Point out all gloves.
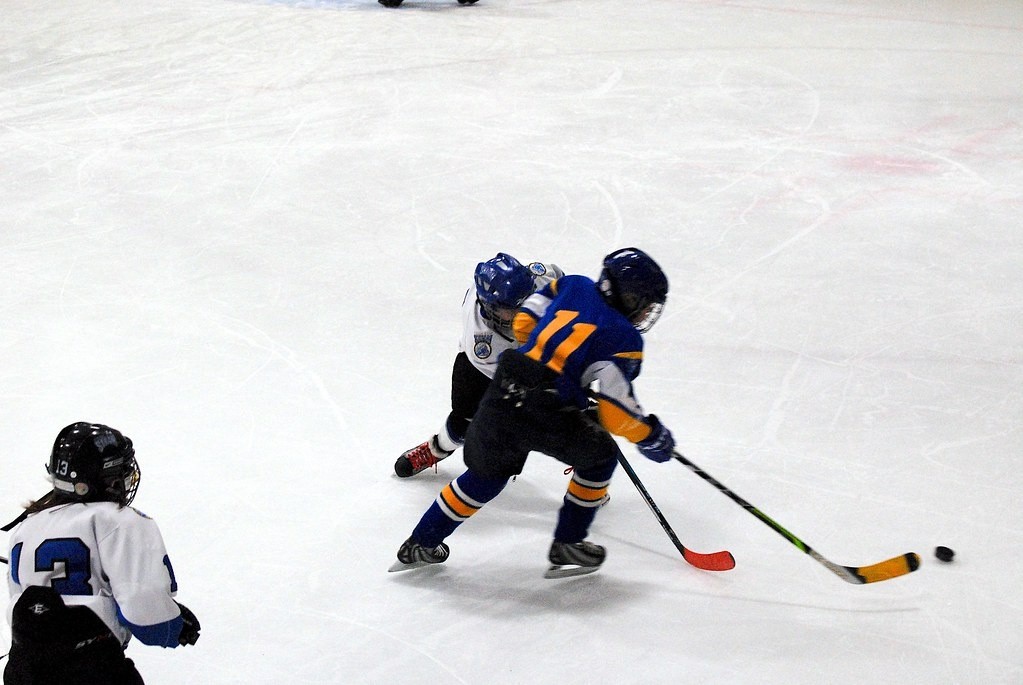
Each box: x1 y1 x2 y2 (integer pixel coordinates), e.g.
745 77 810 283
173 599 201 647
636 414 675 463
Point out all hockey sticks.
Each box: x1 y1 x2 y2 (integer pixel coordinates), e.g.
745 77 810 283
674 450 921 587
619 453 736 572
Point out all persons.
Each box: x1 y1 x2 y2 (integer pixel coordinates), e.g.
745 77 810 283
387 247 675 581
0 421 201 685
395 253 565 478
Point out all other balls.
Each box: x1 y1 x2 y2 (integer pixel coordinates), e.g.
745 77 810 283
935 545 955 562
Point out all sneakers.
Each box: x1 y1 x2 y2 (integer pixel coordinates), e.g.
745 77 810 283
394 434 454 477
388 536 450 571
544 540 606 579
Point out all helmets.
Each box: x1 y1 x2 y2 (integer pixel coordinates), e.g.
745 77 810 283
46 422 141 508
473 253 536 331
602 248 668 335
